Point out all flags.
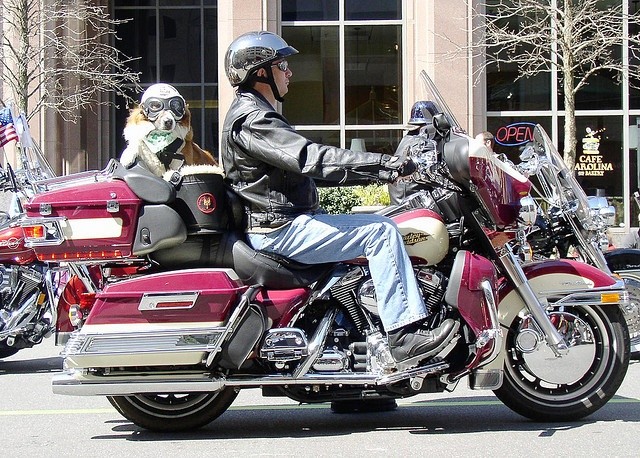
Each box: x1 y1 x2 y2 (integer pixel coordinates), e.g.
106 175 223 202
0 106 19 148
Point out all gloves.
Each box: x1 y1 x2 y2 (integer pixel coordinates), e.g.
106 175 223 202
380 153 416 176
378 170 397 184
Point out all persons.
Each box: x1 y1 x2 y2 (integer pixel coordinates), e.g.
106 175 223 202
221 29 461 414
387 101 445 206
475 132 495 150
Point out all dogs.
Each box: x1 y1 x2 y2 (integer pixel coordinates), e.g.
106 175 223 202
119 83 218 182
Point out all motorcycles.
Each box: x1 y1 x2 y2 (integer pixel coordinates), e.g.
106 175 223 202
510 123 640 360
21 69 631 432
0 160 146 358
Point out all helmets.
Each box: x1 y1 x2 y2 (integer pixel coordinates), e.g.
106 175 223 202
224 31 299 87
141 83 185 104
407 101 442 124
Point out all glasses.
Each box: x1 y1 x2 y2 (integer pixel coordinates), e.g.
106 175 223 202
271 59 289 71
141 96 185 117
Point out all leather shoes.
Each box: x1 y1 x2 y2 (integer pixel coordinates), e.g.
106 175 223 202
388 317 461 372
331 398 398 413
